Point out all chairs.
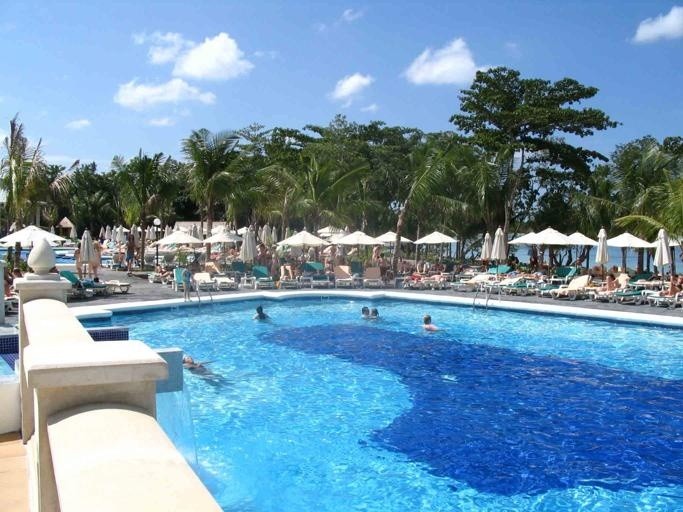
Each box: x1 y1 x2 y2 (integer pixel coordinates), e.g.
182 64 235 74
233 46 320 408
3 270 131 313
148 253 683 310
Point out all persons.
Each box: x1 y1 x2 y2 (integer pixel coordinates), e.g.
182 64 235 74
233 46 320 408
509 254 683 298
359 307 369 320
421 315 440 332
4 236 103 297
181 267 196 302
113 236 466 290
182 353 235 394
367 308 379 319
251 305 270 319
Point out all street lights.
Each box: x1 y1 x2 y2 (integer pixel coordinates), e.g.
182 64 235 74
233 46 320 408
153 217 161 266
